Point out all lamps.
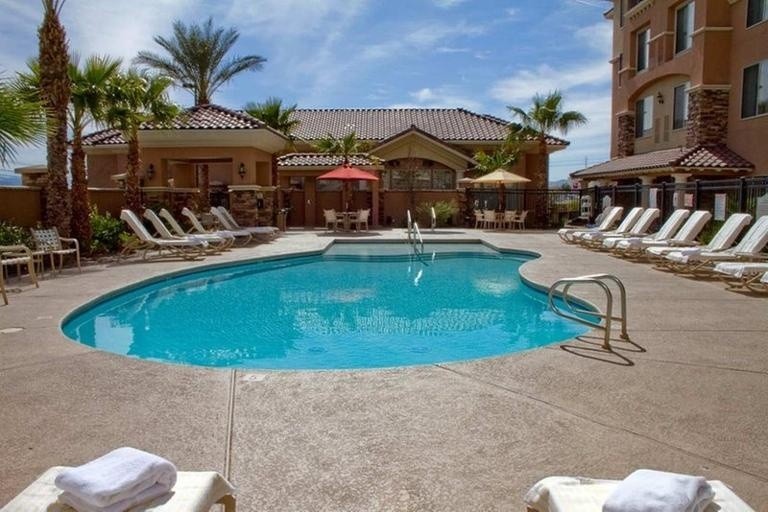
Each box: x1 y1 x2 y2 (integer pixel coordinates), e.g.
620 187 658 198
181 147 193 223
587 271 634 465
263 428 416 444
656 91 664 104
146 163 154 181
239 162 246 179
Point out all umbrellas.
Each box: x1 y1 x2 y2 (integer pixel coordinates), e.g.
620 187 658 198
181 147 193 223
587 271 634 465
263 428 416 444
316 161 380 212
470 168 532 209
471 273 521 298
328 286 371 302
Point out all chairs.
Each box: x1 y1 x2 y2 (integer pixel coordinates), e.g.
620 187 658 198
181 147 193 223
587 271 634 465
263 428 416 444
524 475 759 512
323 208 371 233
0 206 280 305
473 207 530 231
0 465 236 512
557 206 768 295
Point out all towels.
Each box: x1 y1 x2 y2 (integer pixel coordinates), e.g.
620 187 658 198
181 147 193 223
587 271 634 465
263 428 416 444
602 469 721 512
55 446 177 511
